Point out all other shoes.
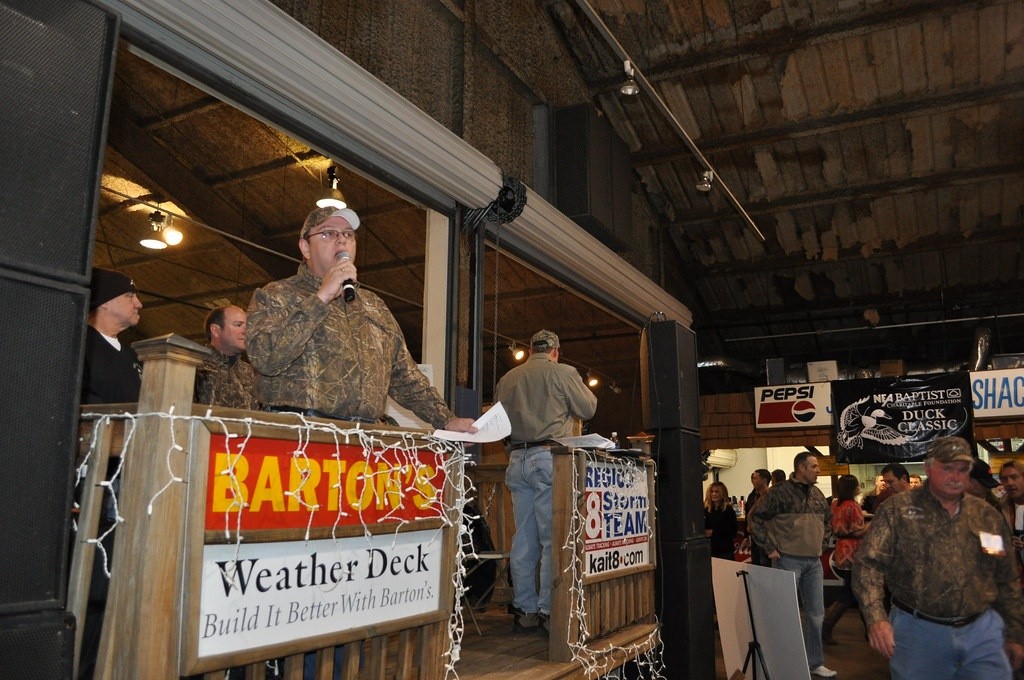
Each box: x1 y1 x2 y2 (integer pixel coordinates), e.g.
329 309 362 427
811 664 837 678
539 614 551 635
514 610 539 633
820 624 840 647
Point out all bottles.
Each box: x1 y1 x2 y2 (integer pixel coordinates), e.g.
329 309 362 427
610 432 620 449
728 496 746 517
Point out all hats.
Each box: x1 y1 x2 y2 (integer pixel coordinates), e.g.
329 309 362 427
90 264 138 310
531 329 561 355
969 459 1001 488
300 206 360 234
930 437 976 464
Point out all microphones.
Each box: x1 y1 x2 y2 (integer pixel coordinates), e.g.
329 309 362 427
334 251 355 301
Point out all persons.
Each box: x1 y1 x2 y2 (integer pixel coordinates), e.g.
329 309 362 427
851 438 1024 680
73 273 144 527
882 463 910 497
967 459 1024 581
705 482 739 560
748 453 837 677
823 474 870 643
910 474 922 487
245 207 478 680
745 469 786 567
497 329 596 636
194 306 261 412
861 474 885 522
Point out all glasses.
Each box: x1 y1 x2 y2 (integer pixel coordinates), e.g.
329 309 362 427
305 230 359 244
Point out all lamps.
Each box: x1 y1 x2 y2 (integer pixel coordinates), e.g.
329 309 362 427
508 341 524 360
696 170 713 191
585 369 598 387
327 165 343 200
609 380 621 393
620 60 639 95
163 214 184 246
140 211 167 250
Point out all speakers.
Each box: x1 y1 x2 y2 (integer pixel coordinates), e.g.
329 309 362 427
647 320 716 680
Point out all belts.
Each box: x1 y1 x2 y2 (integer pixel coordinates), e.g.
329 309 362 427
510 442 561 453
275 404 344 420
891 595 985 628
